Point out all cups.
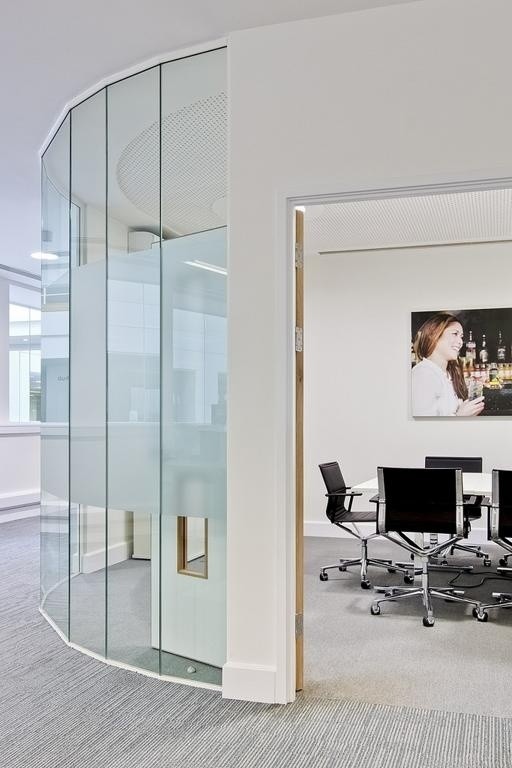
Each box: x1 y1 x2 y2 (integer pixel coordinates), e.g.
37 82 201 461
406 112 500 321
469 377 483 400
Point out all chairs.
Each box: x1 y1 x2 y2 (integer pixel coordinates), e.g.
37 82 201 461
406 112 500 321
319 456 512 626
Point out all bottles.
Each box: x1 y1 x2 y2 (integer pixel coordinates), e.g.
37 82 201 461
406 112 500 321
411 340 416 367
464 329 507 362
460 359 512 388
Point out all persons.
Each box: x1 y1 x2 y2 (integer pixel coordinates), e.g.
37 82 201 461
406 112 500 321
411 313 485 416
